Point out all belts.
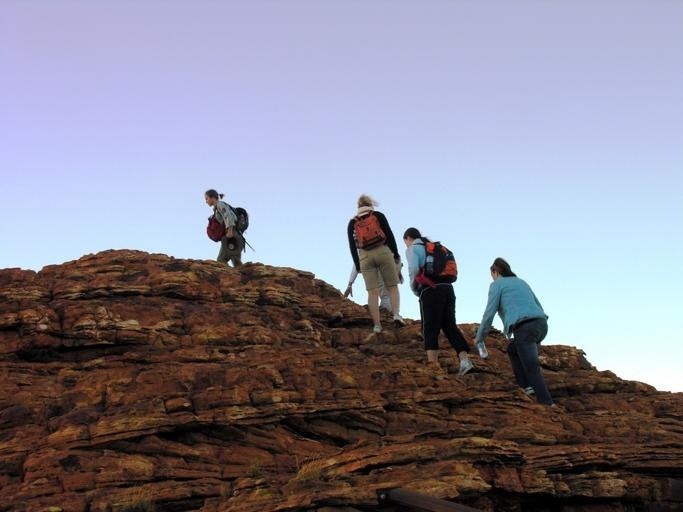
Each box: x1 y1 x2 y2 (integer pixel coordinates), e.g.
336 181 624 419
513 317 540 333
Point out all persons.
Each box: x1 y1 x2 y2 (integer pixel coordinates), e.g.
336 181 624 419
204 189 245 267
402 227 475 377
346 194 408 334
471 256 558 408
341 254 405 314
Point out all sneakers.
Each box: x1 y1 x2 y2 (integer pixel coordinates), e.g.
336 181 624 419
373 325 382 333
524 386 535 395
458 358 474 376
394 316 406 327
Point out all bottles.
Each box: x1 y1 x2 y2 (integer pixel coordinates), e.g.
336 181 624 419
426 254 434 275
477 340 488 358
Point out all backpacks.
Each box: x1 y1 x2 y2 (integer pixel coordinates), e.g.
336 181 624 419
413 241 458 284
206 205 226 242
354 211 386 250
224 202 250 234
